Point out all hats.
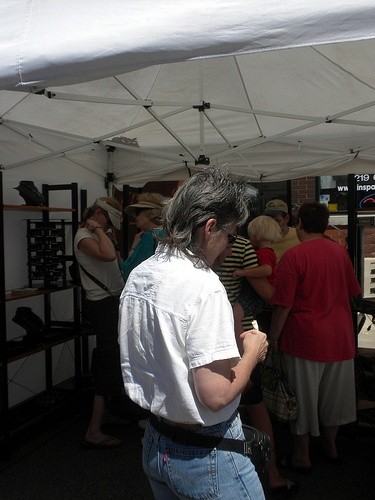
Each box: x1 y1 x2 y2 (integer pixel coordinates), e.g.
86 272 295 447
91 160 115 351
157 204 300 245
130 192 168 209
95 198 124 230
263 199 288 214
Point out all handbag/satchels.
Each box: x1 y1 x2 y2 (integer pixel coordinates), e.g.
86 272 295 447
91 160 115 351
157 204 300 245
261 353 298 428
242 423 276 468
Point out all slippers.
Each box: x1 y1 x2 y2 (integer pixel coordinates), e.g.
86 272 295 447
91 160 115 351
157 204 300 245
270 479 300 500
80 433 125 450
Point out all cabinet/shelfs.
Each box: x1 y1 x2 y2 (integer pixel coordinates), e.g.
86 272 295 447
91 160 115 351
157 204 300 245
0 168 87 463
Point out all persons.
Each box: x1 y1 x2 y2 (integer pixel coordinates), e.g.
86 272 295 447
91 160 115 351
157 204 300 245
117 165 269 500
74 192 362 494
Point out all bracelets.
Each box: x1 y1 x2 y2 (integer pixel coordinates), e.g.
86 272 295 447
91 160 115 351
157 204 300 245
94 226 100 230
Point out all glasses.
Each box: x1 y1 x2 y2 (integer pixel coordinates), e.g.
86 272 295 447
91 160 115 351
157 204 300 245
216 221 237 244
